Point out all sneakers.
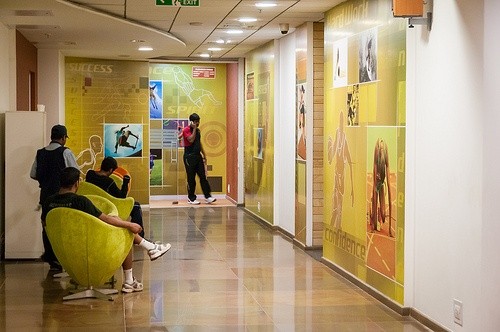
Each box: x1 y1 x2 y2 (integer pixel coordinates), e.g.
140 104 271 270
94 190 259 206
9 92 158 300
148 241 172 260
188 199 200 205
121 276 143 293
204 197 217 204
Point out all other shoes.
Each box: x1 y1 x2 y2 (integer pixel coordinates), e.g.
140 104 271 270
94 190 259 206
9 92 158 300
370 207 372 228
49 262 63 271
378 207 383 229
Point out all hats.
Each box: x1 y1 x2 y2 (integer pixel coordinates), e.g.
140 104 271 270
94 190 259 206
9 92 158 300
51 124 69 139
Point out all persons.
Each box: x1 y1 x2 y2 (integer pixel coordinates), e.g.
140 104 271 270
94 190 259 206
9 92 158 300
113 125 139 153
183 113 216 205
150 84 158 109
150 153 157 174
41 167 171 292
328 112 353 227
370 138 393 237
30 124 80 269
85 157 144 240
298 86 306 144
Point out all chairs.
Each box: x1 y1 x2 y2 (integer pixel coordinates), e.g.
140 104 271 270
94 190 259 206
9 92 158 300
44 166 135 302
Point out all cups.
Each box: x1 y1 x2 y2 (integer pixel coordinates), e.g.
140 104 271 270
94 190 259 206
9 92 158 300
38 104 45 112
